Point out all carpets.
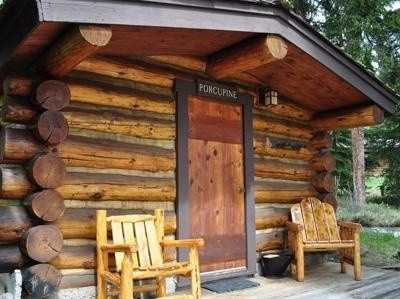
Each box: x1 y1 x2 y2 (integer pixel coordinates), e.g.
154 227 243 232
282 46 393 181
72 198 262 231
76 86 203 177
201 277 260 293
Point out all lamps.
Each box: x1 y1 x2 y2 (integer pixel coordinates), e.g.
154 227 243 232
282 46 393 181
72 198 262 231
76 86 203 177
259 86 278 106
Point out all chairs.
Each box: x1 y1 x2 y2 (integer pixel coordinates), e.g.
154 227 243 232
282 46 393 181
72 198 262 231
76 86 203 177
96 209 205 299
285 197 362 282
